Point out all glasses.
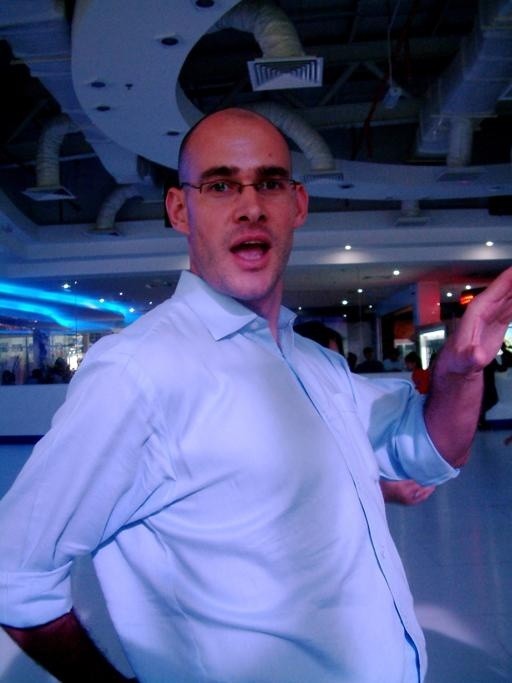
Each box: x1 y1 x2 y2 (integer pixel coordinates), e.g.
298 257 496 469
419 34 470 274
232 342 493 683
177 179 299 199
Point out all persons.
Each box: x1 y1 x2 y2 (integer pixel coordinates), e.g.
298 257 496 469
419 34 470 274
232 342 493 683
340 339 511 449
296 318 437 508
0 355 72 385
1 105 511 683
